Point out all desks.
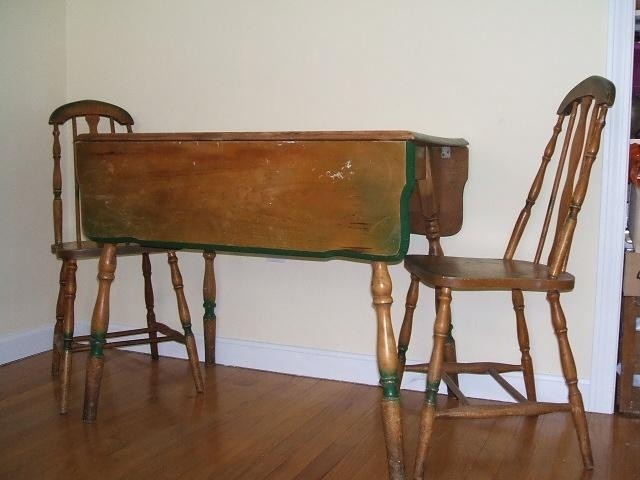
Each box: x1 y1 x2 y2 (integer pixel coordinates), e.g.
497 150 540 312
73 132 470 480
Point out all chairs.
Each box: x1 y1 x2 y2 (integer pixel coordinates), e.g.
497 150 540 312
395 73 617 478
47 98 202 414
